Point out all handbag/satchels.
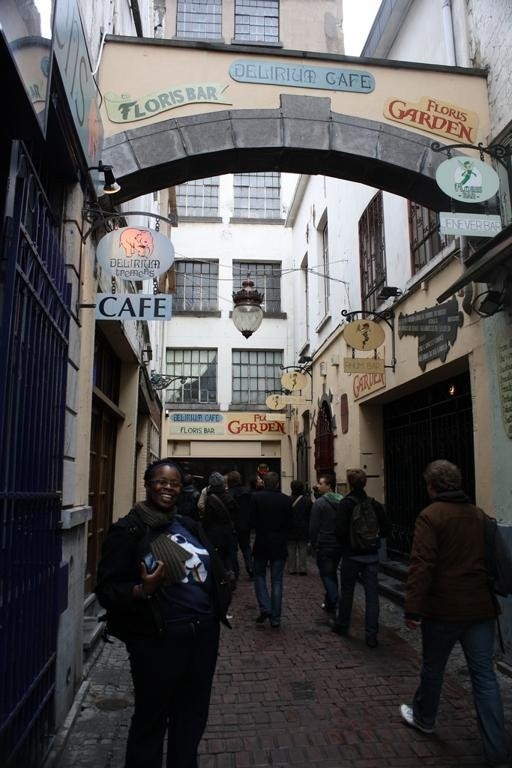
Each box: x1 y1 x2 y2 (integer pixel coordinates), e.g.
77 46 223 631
207 525 233 553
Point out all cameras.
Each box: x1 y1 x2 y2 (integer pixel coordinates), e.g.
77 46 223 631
142 550 158 574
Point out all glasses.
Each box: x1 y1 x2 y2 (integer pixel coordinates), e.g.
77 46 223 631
147 479 183 488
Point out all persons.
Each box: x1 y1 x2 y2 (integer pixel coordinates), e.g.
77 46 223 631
176 460 344 627
94 459 233 768
397 460 510 766
328 468 387 647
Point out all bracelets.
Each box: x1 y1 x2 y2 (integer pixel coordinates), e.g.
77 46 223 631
136 583 154 601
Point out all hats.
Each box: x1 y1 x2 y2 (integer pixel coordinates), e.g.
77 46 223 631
208 472 225 492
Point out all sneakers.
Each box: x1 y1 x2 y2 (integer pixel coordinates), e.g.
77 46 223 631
289 572 308 576
399 703 435 734
256 608 280 628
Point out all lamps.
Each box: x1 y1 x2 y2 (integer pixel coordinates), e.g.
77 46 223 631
231 267 265 339
89 160 121 194
374 283 402 300
471 288 505 317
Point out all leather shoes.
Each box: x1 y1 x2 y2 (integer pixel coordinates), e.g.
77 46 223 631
320 603 378 648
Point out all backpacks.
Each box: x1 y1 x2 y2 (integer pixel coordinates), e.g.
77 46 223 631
486 518 512 598
350 503 381 551
